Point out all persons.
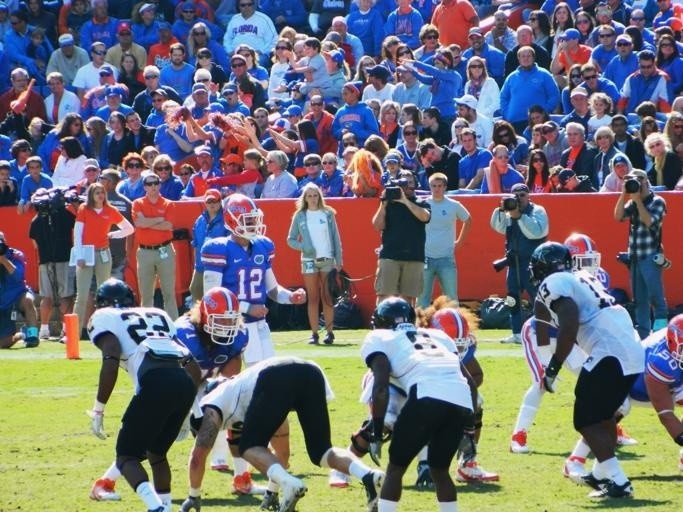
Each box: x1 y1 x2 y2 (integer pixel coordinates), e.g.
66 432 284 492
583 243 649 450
528 241 646 499
0 0 683 348
89 286 268 501
329 296 499 487
361 296 475 512
200 192 307 470
510 233 638 453
175 357 386 512
561 313 683 486
86 278 202 512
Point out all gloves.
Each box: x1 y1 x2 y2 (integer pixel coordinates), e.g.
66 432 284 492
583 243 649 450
456 436 477 466
92 411 107 439
544 367 555 392
369 438 381 465
179 496 201 512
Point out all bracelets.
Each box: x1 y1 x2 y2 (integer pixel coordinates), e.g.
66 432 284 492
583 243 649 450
267 481 279 493
188 487 201 497
93 400 105 412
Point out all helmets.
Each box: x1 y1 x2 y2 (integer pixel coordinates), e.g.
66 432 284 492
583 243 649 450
95 279 134 307
667 314 683 354
201 287 239 324
428 308 470 342
528 234 599 280
224 194 258 229
371 298 416 328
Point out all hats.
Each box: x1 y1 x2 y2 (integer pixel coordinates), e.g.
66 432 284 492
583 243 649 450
205 189 221 202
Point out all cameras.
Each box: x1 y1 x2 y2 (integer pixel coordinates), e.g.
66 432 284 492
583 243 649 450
489 240 517 272
624 174 641 196
503 194 519 209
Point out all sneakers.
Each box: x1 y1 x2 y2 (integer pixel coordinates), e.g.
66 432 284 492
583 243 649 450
90 479 120 500
501 334 521 342
511 430 529 453
23 326 67 347
309 333 334 344
211 458 499 512
565 424 637 498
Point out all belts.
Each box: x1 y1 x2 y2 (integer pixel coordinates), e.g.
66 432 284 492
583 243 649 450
140 240 169 248
317 258 329 261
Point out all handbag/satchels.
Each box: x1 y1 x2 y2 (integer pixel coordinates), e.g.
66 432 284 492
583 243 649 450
482 298 531 329
